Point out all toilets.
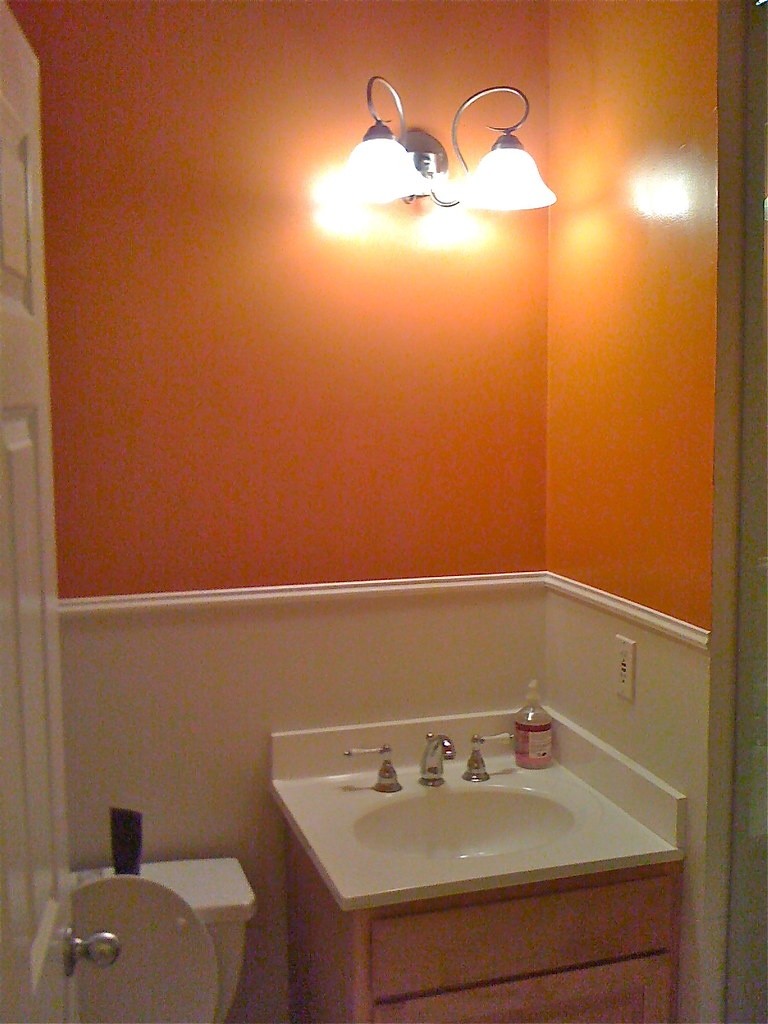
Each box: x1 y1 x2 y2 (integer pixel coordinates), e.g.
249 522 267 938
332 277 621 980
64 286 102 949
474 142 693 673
67 856 257 1024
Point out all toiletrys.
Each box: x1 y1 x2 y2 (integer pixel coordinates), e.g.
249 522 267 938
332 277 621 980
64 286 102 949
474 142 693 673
107 806 143 875
515 677 553 770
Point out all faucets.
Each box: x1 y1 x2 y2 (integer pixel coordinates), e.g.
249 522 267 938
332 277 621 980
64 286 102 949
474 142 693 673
421 730 457 787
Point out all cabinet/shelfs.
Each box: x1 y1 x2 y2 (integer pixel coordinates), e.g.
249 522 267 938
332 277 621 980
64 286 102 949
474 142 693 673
266 704 690 1024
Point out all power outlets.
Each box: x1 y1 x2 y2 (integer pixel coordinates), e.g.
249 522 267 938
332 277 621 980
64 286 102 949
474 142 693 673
611 634 639 703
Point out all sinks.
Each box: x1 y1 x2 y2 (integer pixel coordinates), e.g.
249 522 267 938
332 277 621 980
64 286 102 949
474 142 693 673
350 786 575 857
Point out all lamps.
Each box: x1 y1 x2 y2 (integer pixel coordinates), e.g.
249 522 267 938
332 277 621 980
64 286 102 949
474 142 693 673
342 73 563 217
